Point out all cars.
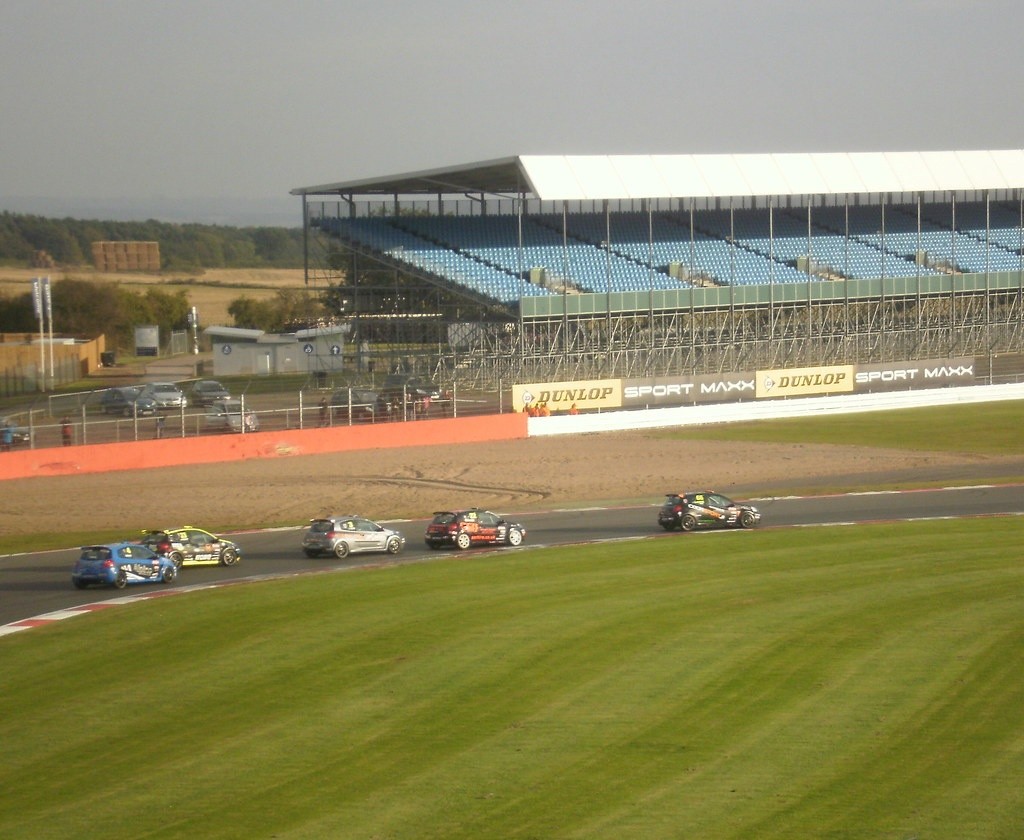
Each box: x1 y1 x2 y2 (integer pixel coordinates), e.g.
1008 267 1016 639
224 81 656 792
379 375 444 411
99 388 156 416
192 381 230 407
0 420 33 444
204 400 261 432
331 390 386 419
143 382 187 409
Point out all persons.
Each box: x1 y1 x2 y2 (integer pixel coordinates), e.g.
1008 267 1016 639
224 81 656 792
0 419 16 452
316 391 452 428
569 403 579 414
59 416 73 445
522 402 551 416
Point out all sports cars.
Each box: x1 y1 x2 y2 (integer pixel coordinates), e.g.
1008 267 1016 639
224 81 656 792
657 490 762 532
424 507 527 551
301 515 406 559
72 542 177 588
137 525 242 571
